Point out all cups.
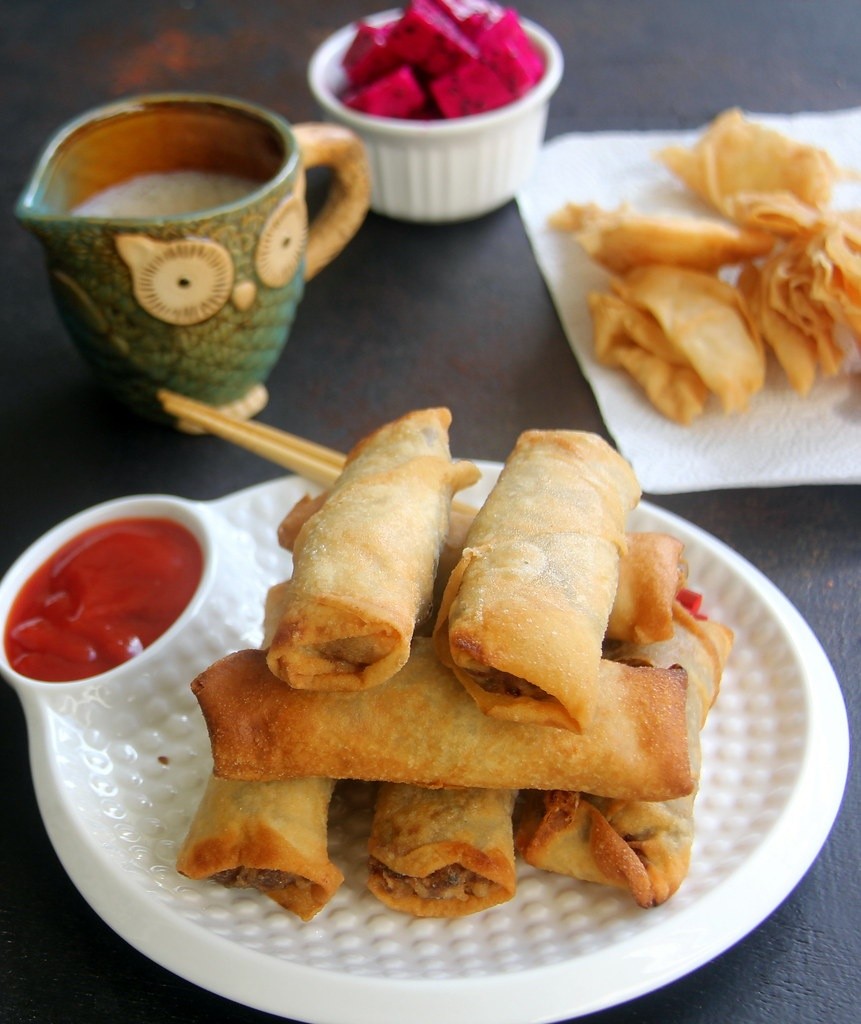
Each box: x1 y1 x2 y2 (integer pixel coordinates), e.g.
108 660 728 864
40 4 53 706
12 92 371 434
0 494 270 742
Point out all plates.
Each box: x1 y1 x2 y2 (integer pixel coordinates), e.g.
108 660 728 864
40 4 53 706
27 458 851 1024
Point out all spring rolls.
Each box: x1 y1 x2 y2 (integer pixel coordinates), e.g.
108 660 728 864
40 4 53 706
176 408 734 920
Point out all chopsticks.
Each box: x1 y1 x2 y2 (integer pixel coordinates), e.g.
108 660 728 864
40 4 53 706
157 389 479 535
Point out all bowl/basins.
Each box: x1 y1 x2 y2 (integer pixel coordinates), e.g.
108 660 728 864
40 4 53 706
306 5 565 224
